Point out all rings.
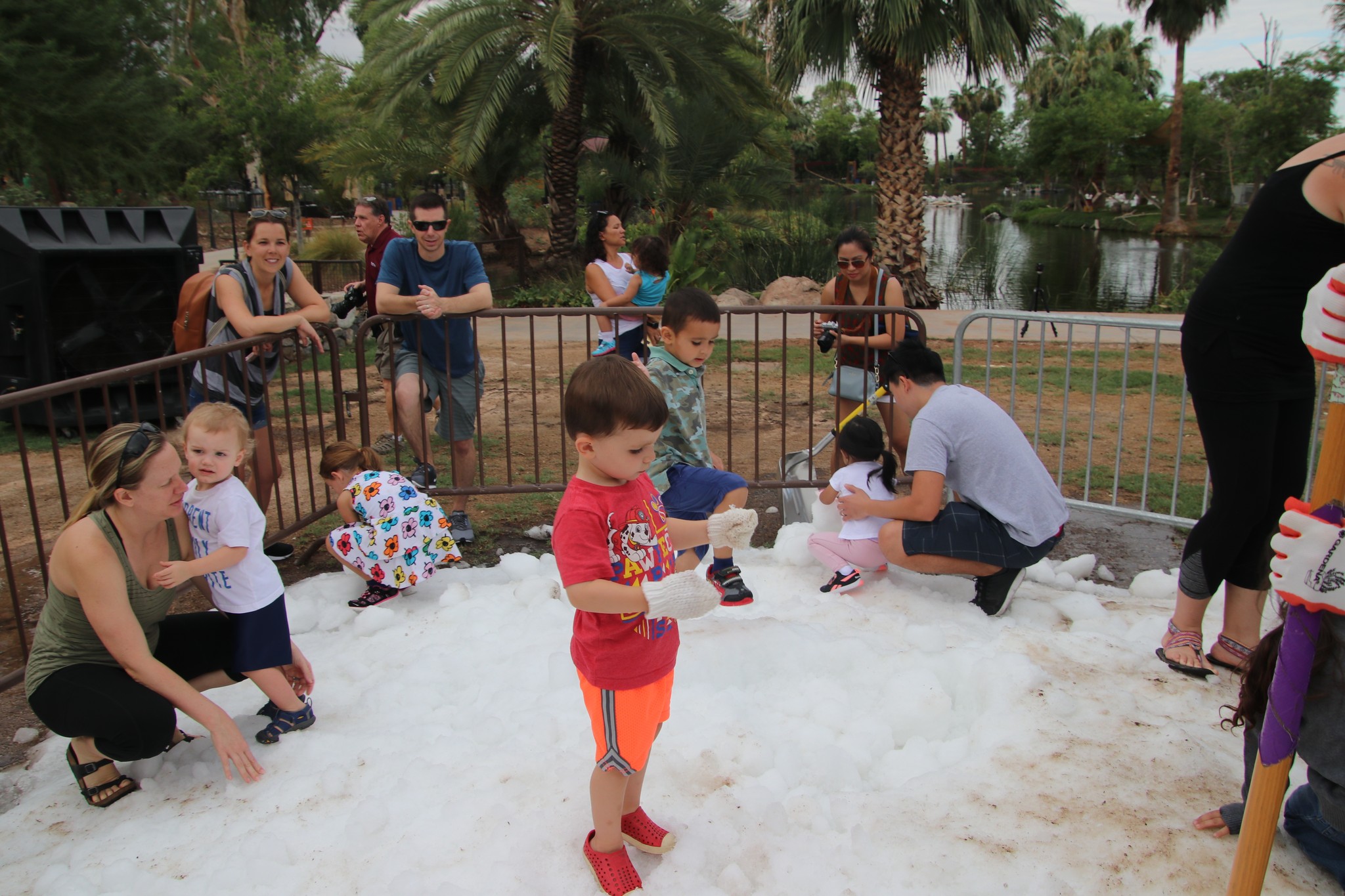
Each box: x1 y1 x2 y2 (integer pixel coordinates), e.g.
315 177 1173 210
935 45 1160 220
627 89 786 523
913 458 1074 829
293 677 300 680
659 336 661 338
841 510 846 516
427 305 429 308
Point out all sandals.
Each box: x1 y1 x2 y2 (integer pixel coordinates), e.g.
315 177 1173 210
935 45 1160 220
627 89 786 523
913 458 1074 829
257 693 305 719
1155 618 1256 677
255 697 315 744
372 433 406 455
582 808 677 896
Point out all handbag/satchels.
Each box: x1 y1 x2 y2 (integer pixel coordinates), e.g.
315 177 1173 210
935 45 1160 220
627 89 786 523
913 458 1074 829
828 364 880 403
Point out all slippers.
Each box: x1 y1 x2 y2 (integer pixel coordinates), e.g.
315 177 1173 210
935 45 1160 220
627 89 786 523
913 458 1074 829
66 742 137 806
163 729 205 752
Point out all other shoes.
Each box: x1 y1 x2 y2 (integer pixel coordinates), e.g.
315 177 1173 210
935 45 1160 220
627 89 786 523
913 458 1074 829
592 340 616 357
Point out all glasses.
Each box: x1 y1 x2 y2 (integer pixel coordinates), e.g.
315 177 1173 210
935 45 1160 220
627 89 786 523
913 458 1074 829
247 210 288 223
411 218 448 232
117 422 161 488
836 252 872 270
363 195 385 216
595 210 610 222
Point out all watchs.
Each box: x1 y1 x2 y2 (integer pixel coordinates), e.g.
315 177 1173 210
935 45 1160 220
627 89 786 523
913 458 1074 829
647 321 658 329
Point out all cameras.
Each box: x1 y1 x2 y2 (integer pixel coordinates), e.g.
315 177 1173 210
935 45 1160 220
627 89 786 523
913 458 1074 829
817 322 839 353
330 284 364 319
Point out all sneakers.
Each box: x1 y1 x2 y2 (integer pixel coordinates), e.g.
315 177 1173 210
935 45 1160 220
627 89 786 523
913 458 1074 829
399 583 417 596
862 561 888 571
262 541 295 560
411 457 438 489
969 564 1026 617
348 583 399 608
447 510 474 543
819 568 862 594
705 565 753 607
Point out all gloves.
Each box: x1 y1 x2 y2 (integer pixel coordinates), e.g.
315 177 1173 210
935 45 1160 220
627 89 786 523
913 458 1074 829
706 506 759 549
641 570 721 620
1269 495 1345 621
1302 264 1345 365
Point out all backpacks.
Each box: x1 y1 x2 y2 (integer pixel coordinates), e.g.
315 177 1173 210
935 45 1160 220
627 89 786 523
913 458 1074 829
172 267 286 359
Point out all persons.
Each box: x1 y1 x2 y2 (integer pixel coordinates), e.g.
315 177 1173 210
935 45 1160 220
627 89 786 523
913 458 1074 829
814 230 913 488
631 286 753 605
1269 261 1345 616
188 214 331 560
591 235 671 356
344 199 441 454
154 402 316 743
1158 135 1345 674
807 417 898 594
376 192 493 543
25 424 314 808
837 339 1069 617
551 355 759 896
581 212 662 362
320 441 462 607
1195 600 1345 890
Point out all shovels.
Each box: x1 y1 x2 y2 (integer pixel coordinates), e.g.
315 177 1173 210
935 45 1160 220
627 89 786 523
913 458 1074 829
777 383 889 527
